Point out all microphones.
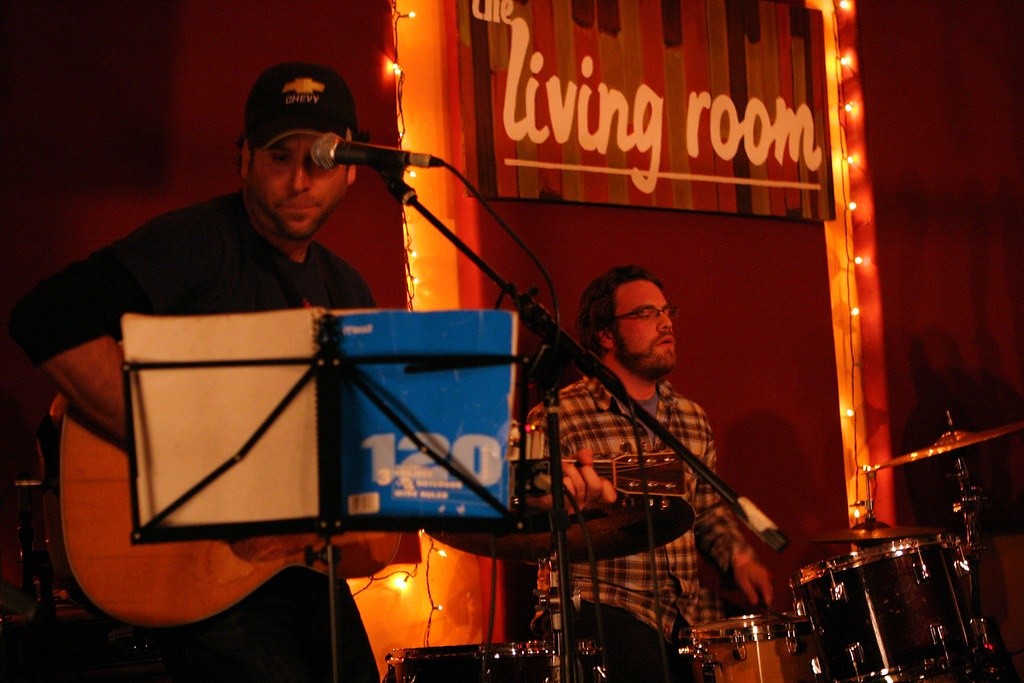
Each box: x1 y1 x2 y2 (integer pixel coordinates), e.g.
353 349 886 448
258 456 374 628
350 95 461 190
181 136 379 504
309 129 443 171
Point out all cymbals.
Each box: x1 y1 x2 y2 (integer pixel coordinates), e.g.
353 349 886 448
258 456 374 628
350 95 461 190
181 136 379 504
812 519 944 546
860 423 1024 475
423 495 696 564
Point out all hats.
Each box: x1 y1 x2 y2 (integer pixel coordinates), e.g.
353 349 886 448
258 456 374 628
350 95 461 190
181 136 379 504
244 62 351 152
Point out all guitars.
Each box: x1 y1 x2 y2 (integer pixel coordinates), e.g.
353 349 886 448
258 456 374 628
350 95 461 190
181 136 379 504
58 335 688 625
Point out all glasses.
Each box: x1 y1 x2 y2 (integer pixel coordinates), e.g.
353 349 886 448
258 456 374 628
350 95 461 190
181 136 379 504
606 305 679 321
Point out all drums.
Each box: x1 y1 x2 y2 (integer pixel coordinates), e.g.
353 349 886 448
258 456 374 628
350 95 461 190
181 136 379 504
385 636 606 683
691 612 816 683
790 534 989 683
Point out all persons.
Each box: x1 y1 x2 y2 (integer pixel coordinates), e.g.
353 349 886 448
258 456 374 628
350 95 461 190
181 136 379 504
9 62 382 683
525 265 773 683
